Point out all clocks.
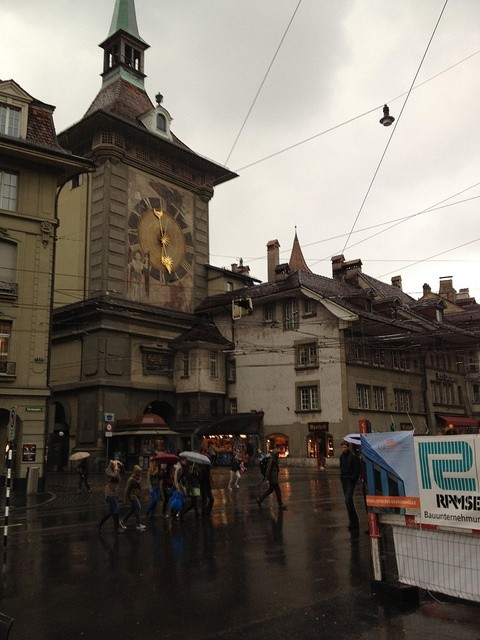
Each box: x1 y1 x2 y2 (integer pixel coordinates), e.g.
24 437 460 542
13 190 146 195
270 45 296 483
127 197 194 282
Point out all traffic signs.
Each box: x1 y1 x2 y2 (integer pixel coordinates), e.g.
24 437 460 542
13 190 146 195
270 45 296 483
7 406 16 442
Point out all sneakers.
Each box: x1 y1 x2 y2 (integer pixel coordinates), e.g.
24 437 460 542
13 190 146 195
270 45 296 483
256 495 262 510
135 523 146 530
227 487 232 491
118 519 128 530
234 483 240 489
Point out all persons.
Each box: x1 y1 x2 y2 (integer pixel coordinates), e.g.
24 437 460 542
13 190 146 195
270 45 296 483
174 456 194 520
159 462 176 518
119 465 146 530
340 441 361 529
183 463 201 520
198 466 214 519
256 449 288 509
145 460 161 520
228 449 243 491
97 459 125 534
78 458 93 492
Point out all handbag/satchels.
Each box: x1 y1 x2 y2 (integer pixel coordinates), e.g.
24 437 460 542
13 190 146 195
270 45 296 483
240 463 248 474
169 489 184 511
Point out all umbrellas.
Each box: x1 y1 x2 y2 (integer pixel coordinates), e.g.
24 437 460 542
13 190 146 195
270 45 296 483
179 451 211 473
151 452 178 468
69 452 91 461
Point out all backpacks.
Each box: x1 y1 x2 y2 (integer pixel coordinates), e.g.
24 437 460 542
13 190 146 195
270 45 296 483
259 455 270 481
